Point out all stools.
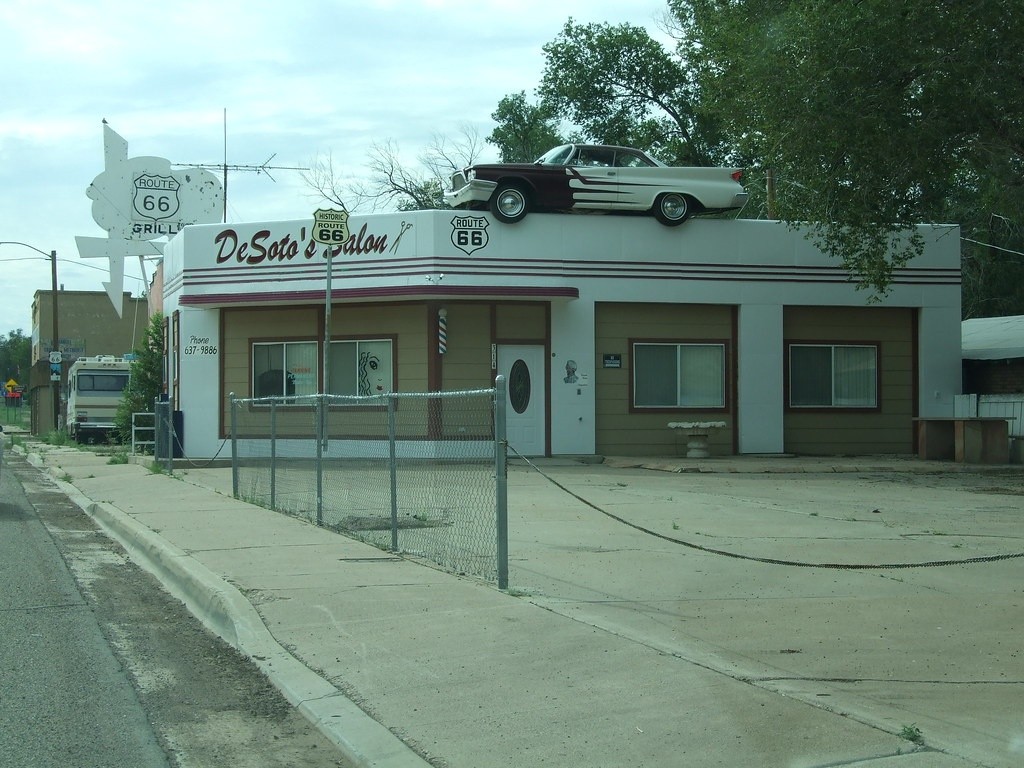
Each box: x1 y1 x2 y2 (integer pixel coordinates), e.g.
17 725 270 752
1009 435 1024 464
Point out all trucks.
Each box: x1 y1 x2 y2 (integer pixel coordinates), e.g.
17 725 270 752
66 354 138 445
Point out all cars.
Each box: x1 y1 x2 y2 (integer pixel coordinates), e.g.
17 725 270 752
441 141 750 223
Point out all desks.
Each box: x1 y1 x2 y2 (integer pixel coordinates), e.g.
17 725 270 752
912 416 1017 464
